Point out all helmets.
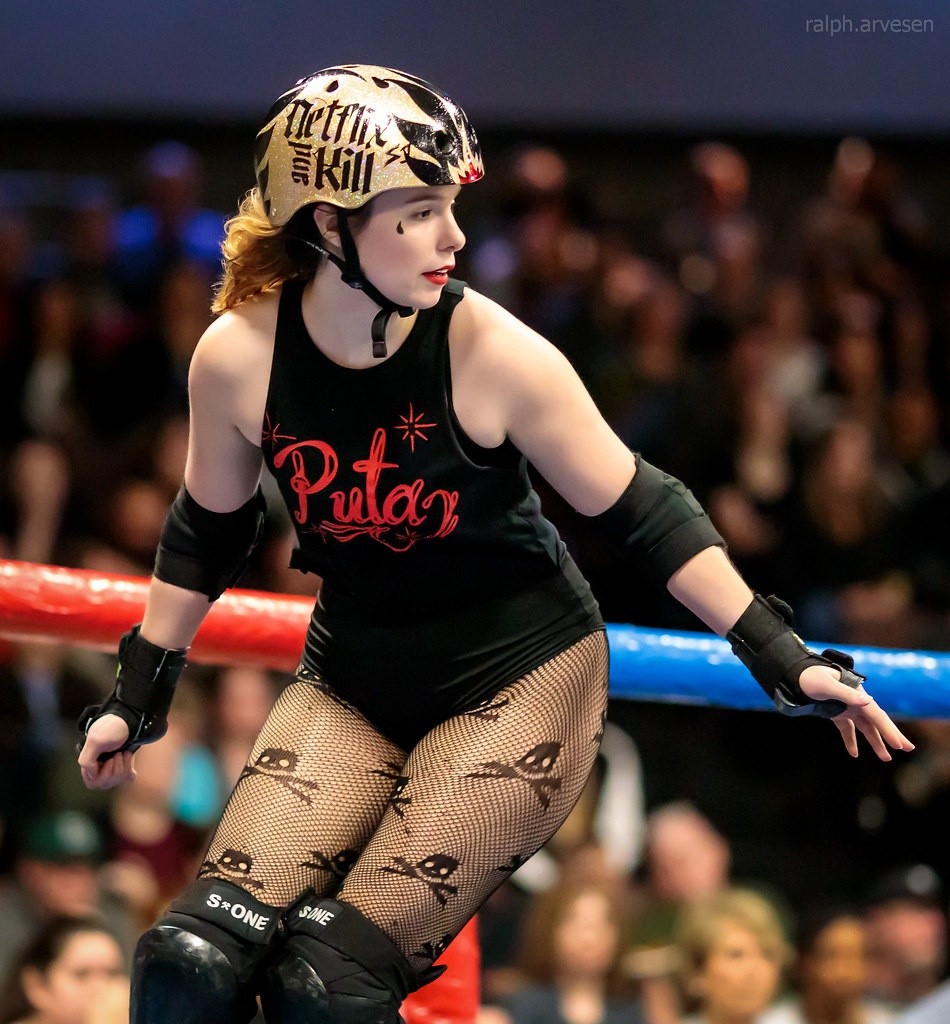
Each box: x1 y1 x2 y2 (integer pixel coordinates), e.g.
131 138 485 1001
253 63 485 239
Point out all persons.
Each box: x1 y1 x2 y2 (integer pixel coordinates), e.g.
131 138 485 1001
0 115 947 1017
70 65 917 1022
